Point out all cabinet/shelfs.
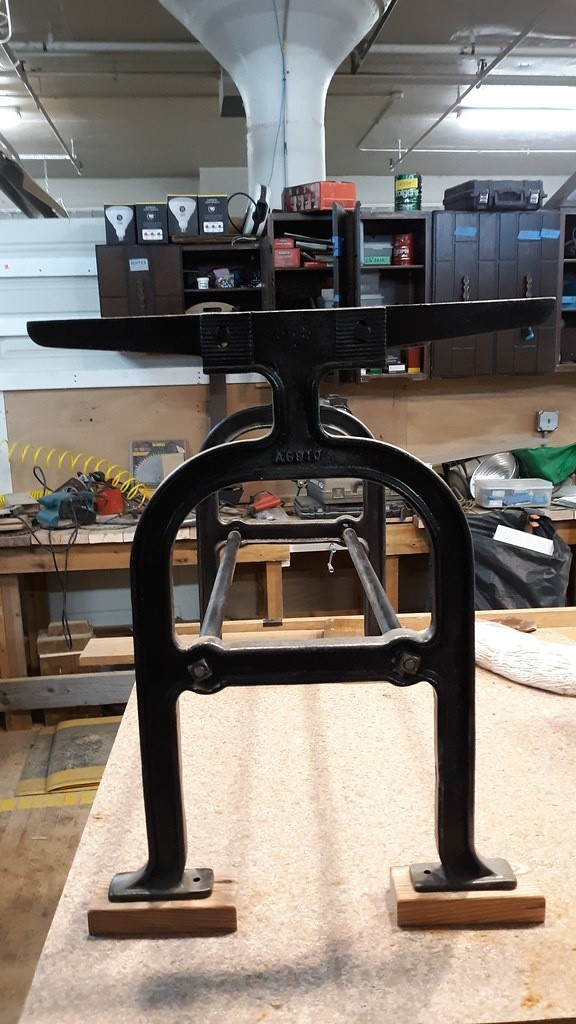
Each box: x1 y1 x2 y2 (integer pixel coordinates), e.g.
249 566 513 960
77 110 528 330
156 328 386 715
95 208 576 383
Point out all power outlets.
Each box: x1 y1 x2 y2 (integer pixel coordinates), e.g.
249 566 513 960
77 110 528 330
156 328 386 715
536 410 559 432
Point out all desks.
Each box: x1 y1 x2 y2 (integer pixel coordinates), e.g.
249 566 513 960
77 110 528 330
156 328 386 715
0 499 576 1024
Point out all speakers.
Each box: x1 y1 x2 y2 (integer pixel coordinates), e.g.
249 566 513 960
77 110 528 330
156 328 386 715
104 193 230 245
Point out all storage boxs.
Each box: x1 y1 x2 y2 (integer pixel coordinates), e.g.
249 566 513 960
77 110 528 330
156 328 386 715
216 274 234 288
363 241 394 266
275 238 294 249
275 248 300 267
361 294 384 306
283 181 356 212
474 478 554 509
104 194 229 247
361 271 380 294
